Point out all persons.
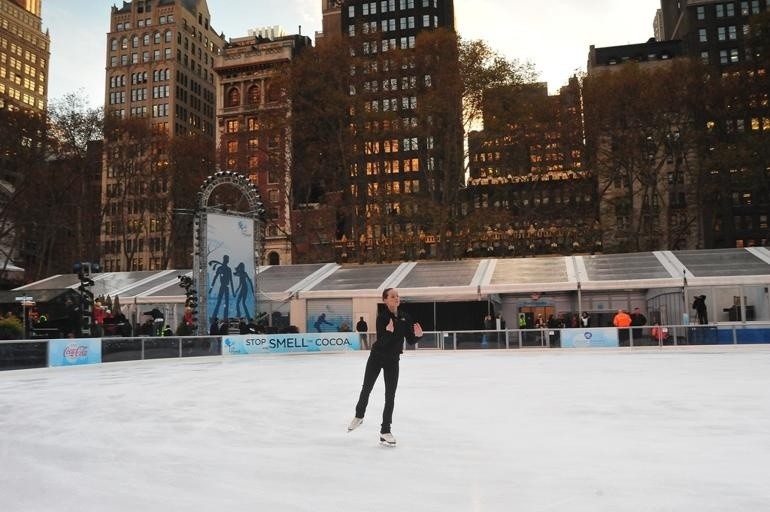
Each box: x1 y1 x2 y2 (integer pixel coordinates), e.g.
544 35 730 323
348 288 424 445
518 311 591 347
208 255 236 322
357 317 369 350
692 294 708 324
139 307 269 337
630 307 647 346
314 313 334 333
233 262 254 324
613 310 632 345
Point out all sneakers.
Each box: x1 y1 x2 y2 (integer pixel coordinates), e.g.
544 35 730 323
379 431 395 444
347 417 362 429
